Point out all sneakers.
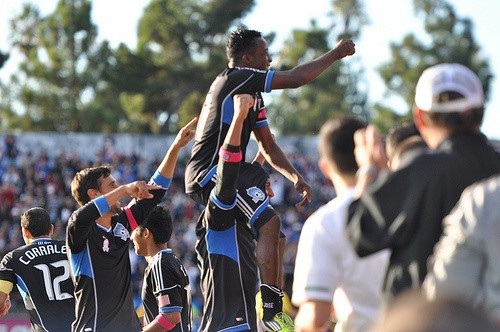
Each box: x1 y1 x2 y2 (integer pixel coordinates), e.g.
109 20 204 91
260 312 294 332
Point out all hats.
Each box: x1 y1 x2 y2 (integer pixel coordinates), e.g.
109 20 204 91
415 61 484 113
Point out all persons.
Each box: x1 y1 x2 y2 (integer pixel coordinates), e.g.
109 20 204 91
184 28 355 332
0 63 500 332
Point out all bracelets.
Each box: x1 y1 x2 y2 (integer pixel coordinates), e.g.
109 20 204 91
356 166 378 178
